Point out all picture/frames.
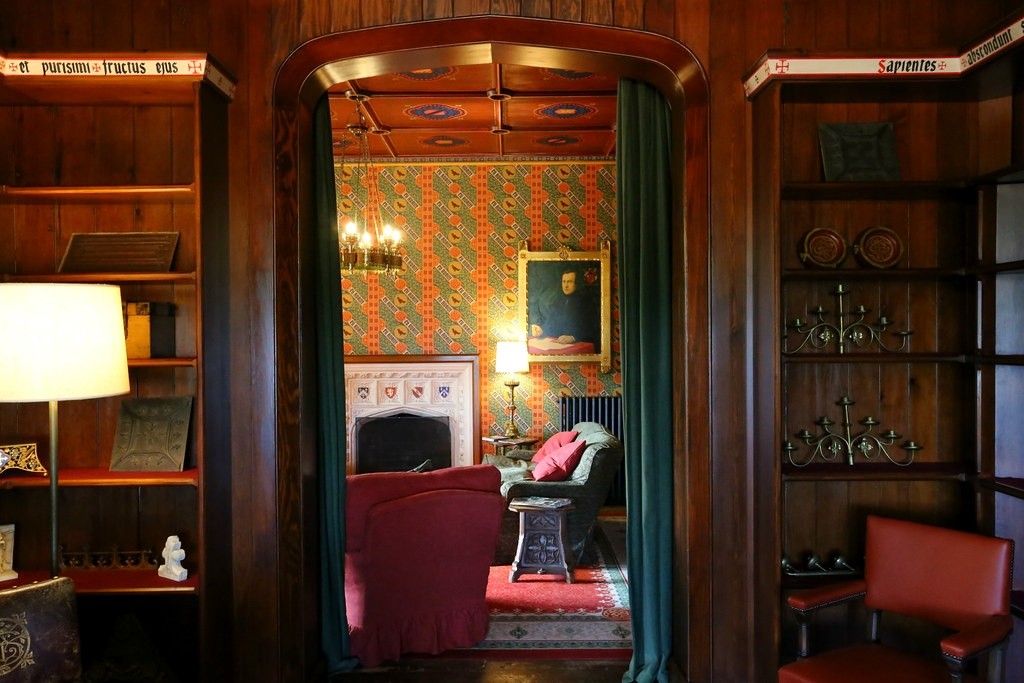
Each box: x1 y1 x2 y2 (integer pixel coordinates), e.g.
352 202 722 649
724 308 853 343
518 239 610 373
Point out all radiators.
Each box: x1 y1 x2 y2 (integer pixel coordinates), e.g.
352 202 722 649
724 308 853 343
556 395 626 506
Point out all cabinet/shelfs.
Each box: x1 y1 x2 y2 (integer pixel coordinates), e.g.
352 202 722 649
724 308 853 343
747 20 1024 682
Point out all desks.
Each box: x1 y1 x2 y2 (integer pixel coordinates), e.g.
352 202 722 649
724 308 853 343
507 497 577 585
481 436 539 456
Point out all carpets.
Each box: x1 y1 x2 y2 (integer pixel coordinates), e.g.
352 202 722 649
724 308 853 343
406 524 634 663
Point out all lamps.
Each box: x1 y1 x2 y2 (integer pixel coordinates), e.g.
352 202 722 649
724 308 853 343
330 89 404 274
0 283 130 576
495 340 529 439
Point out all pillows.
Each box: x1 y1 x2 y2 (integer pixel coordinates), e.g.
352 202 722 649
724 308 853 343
534 439 586 480
531 431 578 463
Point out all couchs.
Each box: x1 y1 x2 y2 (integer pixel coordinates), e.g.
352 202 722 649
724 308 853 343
478 421 621 566
340 464 504 667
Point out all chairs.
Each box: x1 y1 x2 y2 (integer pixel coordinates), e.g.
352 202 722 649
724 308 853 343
0 53 233 683
778 513 1016 683
